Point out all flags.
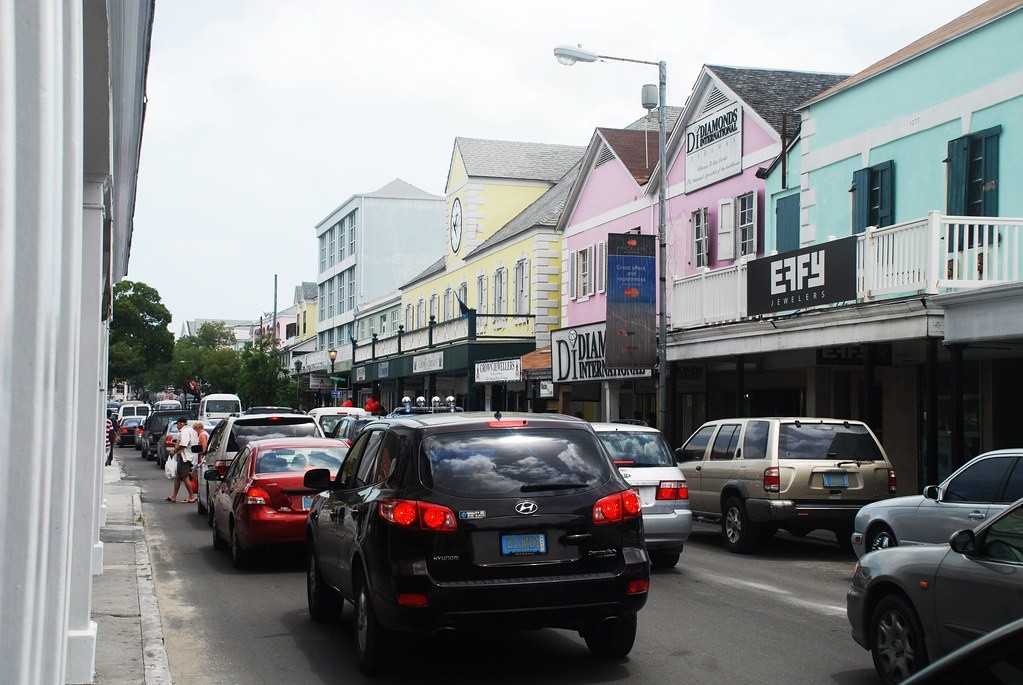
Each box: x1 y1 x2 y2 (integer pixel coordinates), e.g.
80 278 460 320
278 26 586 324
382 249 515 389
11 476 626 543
458 298 469 318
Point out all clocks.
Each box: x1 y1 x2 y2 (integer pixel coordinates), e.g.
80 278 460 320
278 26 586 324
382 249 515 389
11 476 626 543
450 197 464 253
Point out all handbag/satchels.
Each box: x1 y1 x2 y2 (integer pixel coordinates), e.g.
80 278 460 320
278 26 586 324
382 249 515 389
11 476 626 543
165 455 177 480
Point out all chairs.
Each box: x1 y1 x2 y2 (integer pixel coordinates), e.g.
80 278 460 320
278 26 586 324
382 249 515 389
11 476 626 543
259 457 286 474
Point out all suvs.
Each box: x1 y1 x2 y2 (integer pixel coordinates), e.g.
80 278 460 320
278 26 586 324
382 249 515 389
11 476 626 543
675 417 896 553
303 409 650 674
587 419 693 568
191 415 328 526
139 410 198 461
243 406 294 415
332 414 386 441
106 402 119 419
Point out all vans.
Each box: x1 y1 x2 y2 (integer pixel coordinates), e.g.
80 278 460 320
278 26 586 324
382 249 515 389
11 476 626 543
198 394 242 420
308 407 365 436
154 401 182 410
117 402 151 420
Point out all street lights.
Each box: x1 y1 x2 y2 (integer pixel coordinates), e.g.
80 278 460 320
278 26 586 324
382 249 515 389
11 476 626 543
223 316 263 350
294 359 302 408
328 348 337 407
554 46 666 431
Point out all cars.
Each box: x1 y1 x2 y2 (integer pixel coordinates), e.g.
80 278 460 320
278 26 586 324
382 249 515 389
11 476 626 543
118 417 145 448
204 436 352 569
848 447 1023 685
157 419 223 469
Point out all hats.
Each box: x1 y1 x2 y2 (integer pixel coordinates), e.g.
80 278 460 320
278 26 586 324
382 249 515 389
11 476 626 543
174 417 187 424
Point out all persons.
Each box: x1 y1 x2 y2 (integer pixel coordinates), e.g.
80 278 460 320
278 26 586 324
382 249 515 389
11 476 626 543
105 409 120 466
372 400 388 416
191 421 210 491
165 417 198 504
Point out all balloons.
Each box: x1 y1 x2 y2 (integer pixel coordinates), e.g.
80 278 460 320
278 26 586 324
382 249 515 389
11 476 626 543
365 398 377 411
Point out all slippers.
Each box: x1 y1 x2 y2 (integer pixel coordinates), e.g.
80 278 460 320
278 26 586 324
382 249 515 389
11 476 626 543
164 496 177 503
181 499 195 503
